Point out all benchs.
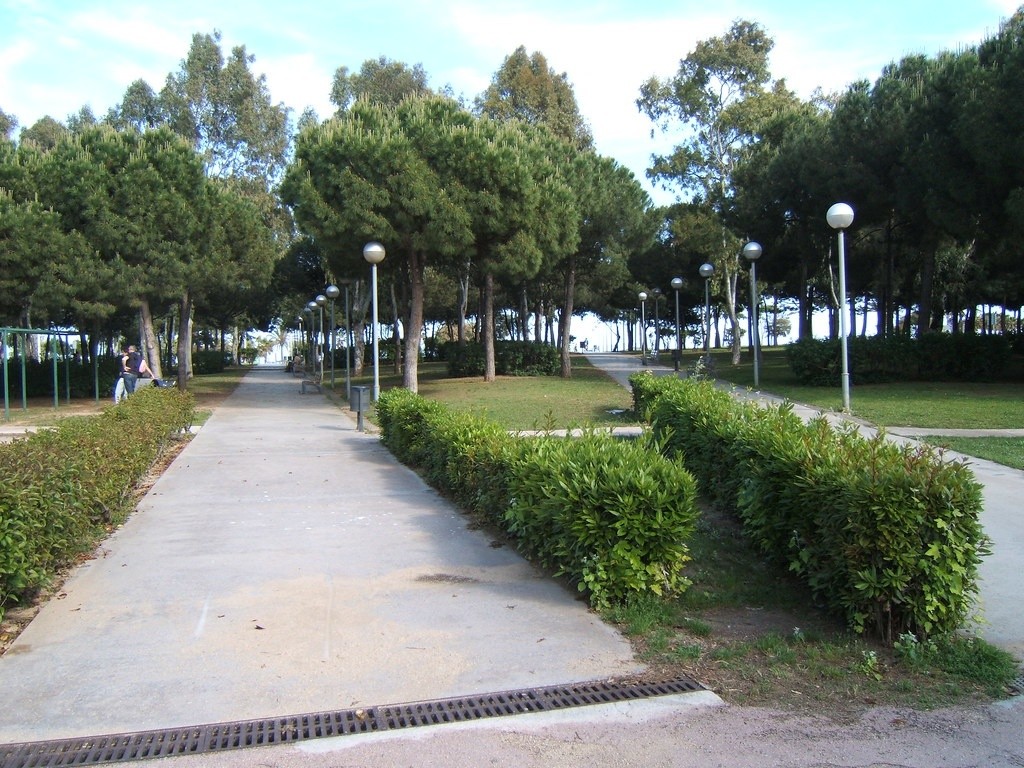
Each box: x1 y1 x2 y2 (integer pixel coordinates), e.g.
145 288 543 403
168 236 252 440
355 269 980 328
292 360 307 378
641 349 660 366
685 356 719 382
301 371 323 395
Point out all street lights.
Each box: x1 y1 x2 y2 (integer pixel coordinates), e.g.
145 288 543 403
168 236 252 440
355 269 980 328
309 300 318 375
670 277 682 368
825 203 855 416
326 284 340 390
316 295 326 383
638 293 647 360
700 263 713 366
652 287 662 364
743 241 762 390
363 240 386 401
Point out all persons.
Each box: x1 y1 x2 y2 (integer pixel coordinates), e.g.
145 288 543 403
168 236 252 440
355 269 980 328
284 354 304 372
121 345 154 399
114 347 130 404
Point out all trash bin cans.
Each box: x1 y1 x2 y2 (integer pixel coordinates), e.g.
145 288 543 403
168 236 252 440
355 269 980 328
349 385 371 431
